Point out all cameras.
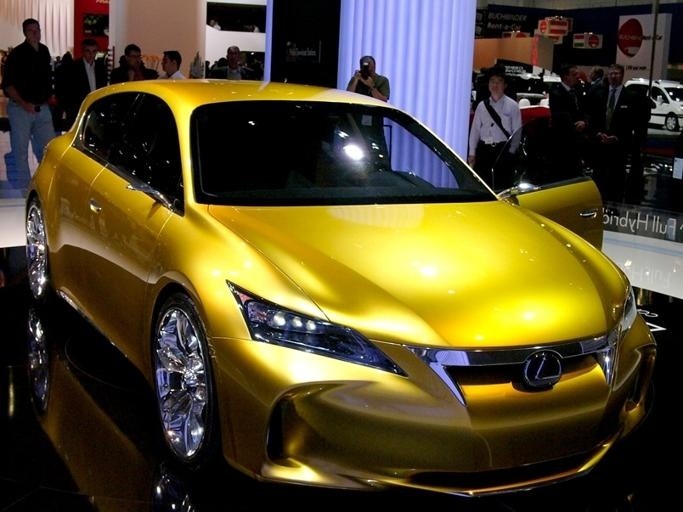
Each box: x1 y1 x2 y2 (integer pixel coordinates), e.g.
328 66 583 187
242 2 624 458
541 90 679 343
359 62 370 81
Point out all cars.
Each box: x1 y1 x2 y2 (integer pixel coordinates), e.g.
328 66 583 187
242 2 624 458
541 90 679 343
623 77 683 132
24 76 656 498
6 297 230 512
471 68 567 111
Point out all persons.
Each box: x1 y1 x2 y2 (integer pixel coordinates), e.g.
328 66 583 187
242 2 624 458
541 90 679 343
344 55 391 171
467 75 523 188
202 46 263 79
108 44 157 85
214 21 220 30
153 51 187 80
551 62 658 200
1 17 61 189
60 39 105 131
241 25 260 33
209 19 215 26
61 52 70 62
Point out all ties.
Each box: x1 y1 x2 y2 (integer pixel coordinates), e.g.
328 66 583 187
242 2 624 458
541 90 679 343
606 87 618 117
569 89 577 108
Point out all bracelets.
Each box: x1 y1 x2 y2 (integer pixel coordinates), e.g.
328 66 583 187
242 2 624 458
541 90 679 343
369 85 376 93
